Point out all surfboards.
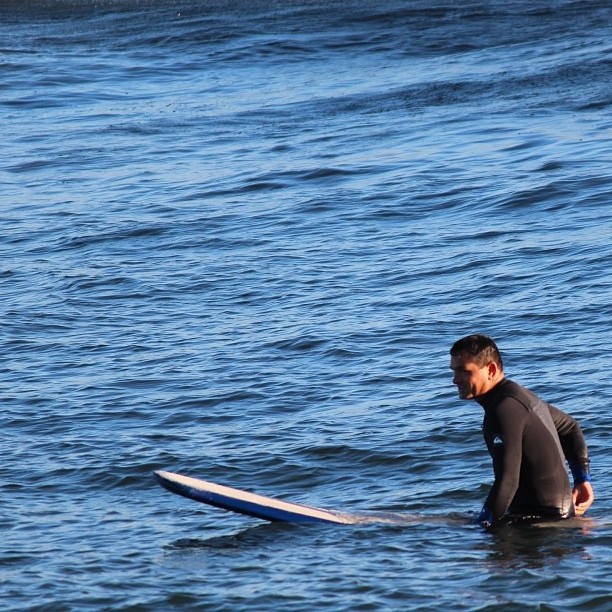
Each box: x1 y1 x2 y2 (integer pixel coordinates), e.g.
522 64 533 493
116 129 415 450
153 468 358 526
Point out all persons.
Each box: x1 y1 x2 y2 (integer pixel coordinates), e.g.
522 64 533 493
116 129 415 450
449 334 594 530
